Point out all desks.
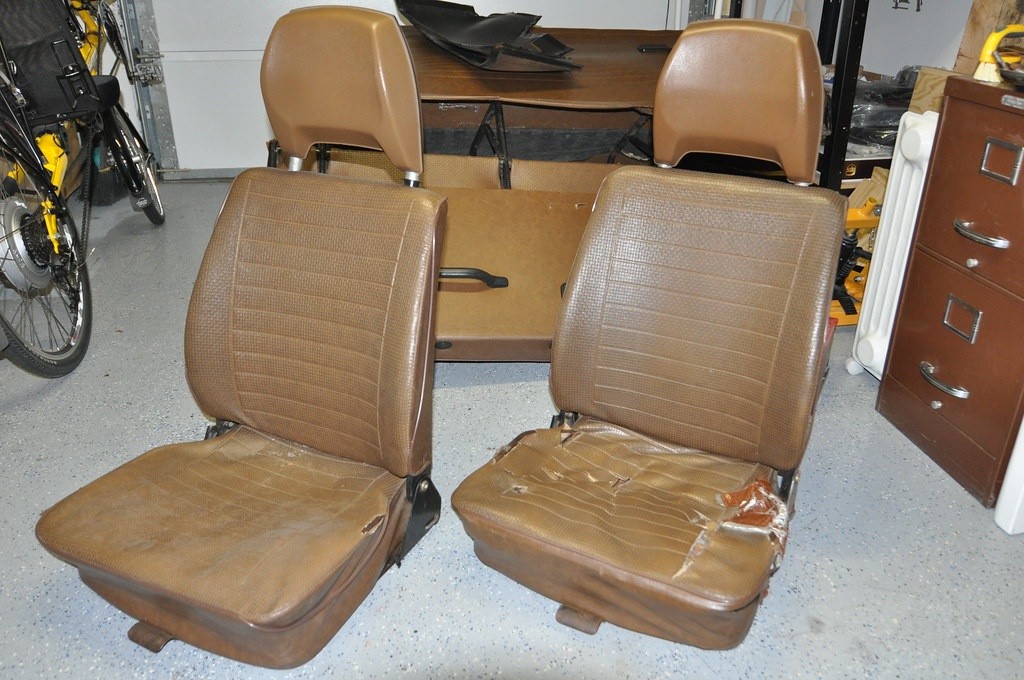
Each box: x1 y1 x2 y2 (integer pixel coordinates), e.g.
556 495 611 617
401 26 683 190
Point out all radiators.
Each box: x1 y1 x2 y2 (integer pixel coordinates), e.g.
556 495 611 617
846 110 940 381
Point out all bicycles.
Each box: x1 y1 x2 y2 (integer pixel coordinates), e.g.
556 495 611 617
0 0 166 379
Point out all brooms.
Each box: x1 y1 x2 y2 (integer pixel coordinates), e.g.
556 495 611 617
72 10 130 208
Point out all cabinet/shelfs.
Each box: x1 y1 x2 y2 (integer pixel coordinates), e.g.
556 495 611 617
874 76 1024 508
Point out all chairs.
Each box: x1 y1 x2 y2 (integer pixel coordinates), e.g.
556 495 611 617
34 6 449 668
451 18 850 649
